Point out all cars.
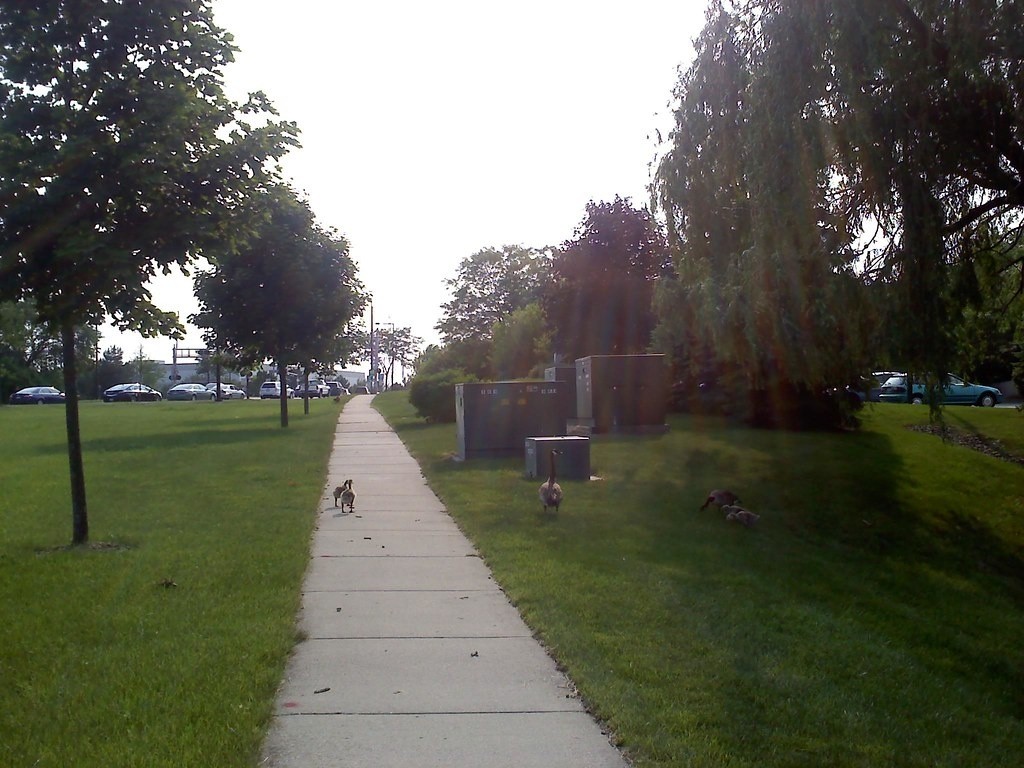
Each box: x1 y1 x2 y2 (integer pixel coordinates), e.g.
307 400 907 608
166 383 217 401
206 382 246 400
103 383 163 403
824 370 902 402
879 372 1003 408
8 386 66 405
294 378 371 399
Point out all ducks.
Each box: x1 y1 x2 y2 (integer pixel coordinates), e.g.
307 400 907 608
340 479 355 513
332 480 354 507
720 504 745 514
699 489 742 513
727 512 760 528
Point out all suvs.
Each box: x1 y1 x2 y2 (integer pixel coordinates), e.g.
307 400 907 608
258 380 296 399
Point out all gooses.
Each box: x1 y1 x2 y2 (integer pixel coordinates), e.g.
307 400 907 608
538 449 563 512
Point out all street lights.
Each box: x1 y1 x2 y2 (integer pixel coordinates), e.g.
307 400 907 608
375 322 394 386
